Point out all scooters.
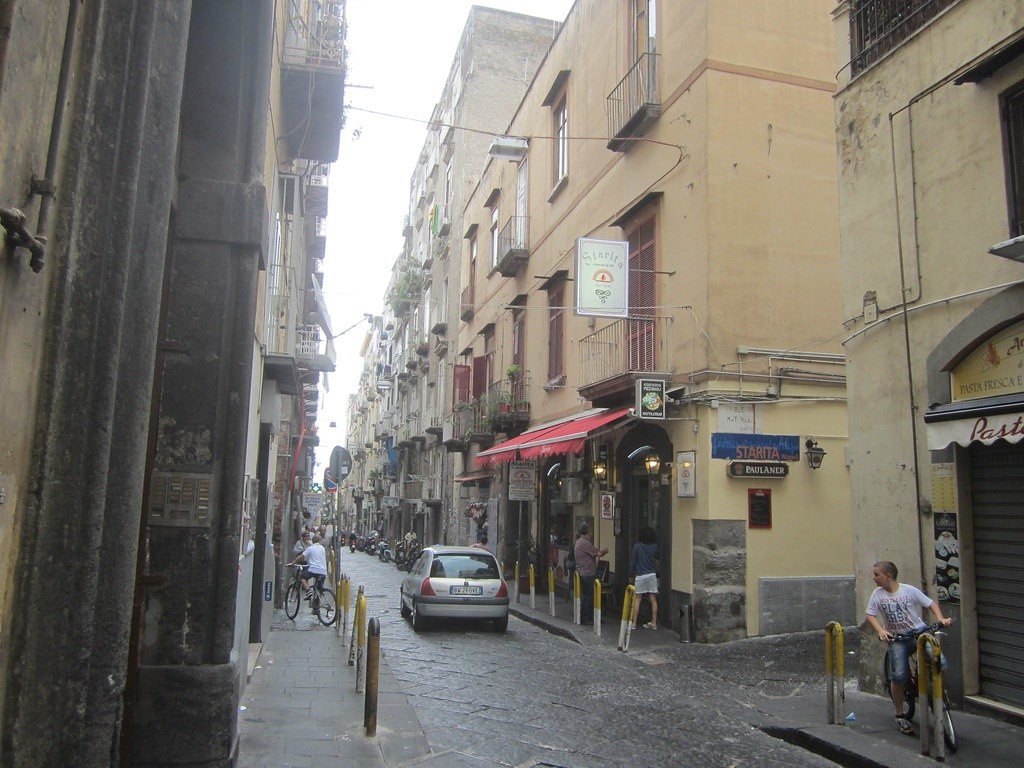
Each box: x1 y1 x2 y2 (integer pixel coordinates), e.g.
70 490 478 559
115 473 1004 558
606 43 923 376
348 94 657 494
339 528 420 574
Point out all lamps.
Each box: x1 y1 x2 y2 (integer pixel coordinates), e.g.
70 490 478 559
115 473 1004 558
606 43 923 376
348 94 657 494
627 407 638 419
804 439 828 471
593 452 615 481
642 445 672 482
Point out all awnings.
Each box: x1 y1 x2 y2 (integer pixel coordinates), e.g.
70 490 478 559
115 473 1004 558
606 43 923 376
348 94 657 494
474 405 636 466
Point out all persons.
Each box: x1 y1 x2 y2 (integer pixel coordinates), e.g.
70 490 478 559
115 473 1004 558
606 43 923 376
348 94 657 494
370 528 379 541
405 529 416 545
349 530 356 543
568 524 608 621
865 561 950 733
469 535 490 551
293 524 331 589
628 526 659 630
287 535 327 615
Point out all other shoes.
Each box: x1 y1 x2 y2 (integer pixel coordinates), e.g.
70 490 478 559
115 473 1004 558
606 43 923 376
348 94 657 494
582 616 596 625
303 590 314 600
628 624 636 629
311 610 320 614
895 717 913 732
643 622 658 629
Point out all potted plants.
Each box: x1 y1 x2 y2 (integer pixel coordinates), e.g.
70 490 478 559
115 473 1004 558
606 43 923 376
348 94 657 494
512 532 540 594
517 400 531 413
499 393 514 415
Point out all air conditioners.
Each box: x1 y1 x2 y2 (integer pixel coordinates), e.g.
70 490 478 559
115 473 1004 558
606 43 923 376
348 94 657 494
559 476 584 503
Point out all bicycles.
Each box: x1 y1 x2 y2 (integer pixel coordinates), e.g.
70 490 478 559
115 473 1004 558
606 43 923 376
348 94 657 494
879 618 960 753
283 563 339 626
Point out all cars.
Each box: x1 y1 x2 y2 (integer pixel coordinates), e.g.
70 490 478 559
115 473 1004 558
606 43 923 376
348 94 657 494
396 545 510 632
